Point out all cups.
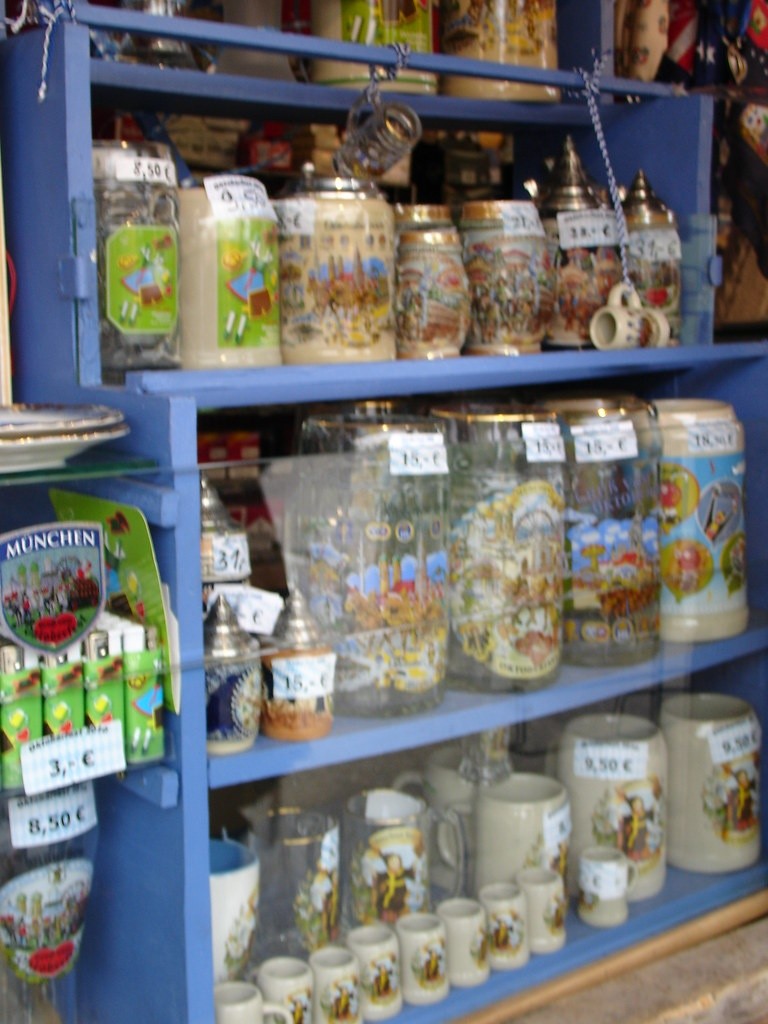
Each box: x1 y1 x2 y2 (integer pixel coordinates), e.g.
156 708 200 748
332 85 423 185
309 0 564 103
588 282 673 351
294 396 751 717
207 693 764 1023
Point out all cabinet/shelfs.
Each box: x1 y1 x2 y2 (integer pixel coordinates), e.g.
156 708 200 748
0 0 768 1024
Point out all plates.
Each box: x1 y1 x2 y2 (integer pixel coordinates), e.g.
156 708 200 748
0 404 132 477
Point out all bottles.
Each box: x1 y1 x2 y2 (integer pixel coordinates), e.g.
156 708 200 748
91 132 687 386
200 468 340 754
